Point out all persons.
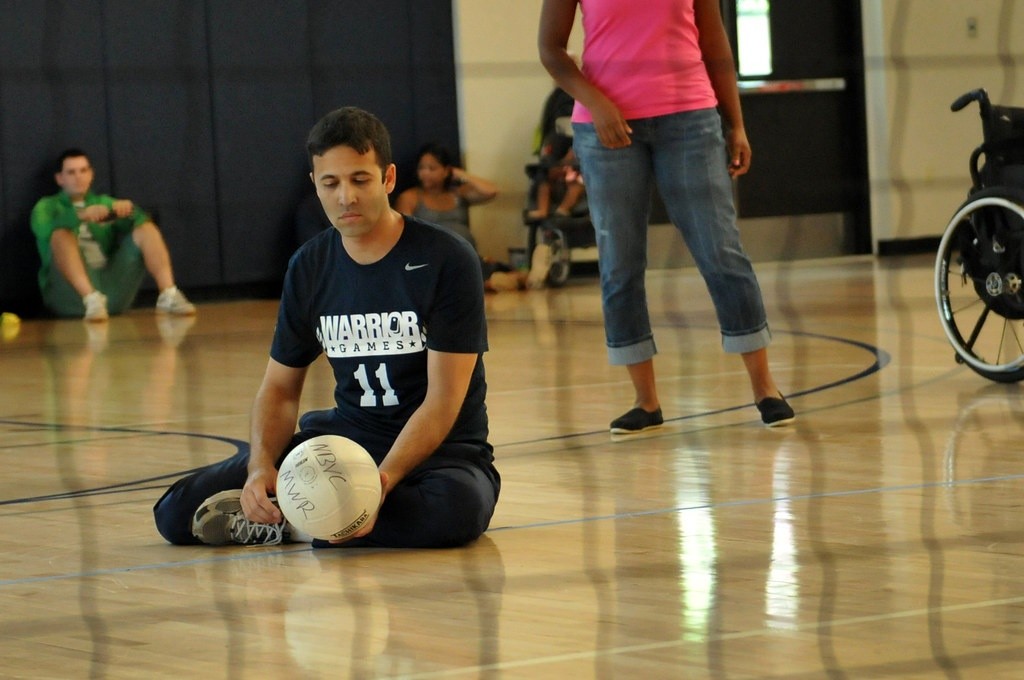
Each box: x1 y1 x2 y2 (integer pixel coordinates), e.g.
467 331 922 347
30 149 197 320
526 134 583 218
394 146 552 293
152 106 501 549
536 0 796 435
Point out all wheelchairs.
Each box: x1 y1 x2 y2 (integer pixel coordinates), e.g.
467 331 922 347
933 87 1024 385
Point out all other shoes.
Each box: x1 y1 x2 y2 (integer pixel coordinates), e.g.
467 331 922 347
525 243 553 290
609 405 664 434
754 390 796 427
490 270 523 292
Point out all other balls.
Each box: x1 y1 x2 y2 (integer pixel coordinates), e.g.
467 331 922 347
276 435 382 550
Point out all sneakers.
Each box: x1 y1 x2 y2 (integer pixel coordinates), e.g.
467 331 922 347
191 488 288 546
156 288 195 315
82 289 110 321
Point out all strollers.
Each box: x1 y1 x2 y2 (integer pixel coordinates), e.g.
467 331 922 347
522 88 597 286
